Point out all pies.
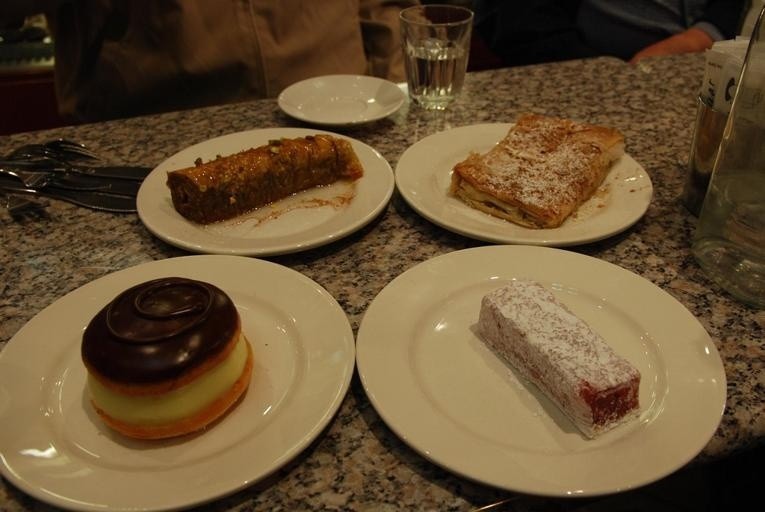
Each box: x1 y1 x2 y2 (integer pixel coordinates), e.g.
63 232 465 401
450 115 625 229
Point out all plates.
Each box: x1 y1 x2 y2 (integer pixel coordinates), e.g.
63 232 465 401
1 253 356 512
356 244 729 499
277 73 406 126
135 128 395 256
395 122 653 248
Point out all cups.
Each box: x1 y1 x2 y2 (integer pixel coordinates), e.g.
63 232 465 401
398 5 474 111
680 91 729 220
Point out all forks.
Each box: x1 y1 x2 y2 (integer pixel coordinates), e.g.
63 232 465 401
0 139 155 214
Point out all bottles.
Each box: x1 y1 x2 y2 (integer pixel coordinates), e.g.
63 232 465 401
691 4 764 311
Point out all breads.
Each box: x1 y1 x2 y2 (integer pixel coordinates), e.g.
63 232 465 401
168 135 363 225
479 279 640 438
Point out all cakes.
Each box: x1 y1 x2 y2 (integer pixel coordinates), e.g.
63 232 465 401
81 279 254 440
